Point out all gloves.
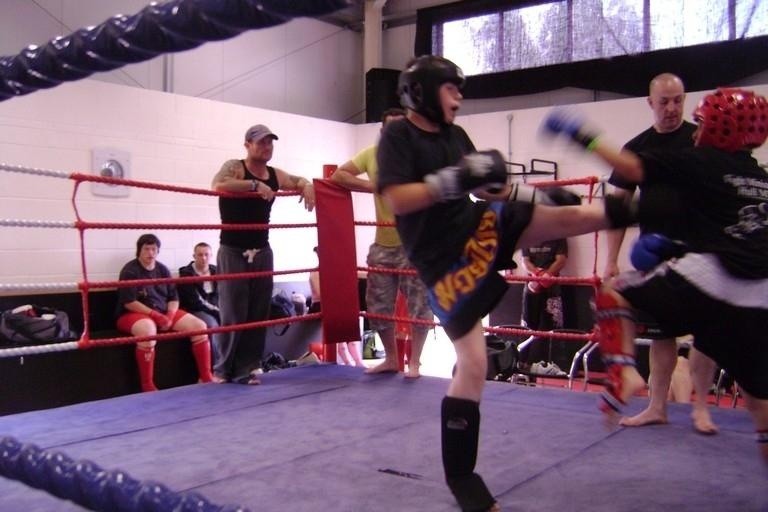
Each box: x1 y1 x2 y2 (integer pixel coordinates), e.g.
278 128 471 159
509 180 584 208
529 102 608 156
422 145 510 206
627 232 688 275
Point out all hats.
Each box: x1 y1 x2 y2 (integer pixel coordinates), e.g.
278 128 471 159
244 124 280 145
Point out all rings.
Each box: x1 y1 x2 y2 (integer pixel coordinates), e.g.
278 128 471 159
311 200 314 203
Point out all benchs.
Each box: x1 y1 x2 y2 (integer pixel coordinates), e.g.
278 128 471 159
0 280 363 416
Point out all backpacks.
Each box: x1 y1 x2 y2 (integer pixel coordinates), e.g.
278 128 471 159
271 286 298 337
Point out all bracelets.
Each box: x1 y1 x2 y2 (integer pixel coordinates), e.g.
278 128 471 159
256 181 260 189
252 180 256 192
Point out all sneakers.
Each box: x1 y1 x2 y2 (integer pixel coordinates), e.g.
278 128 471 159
530 358 567 379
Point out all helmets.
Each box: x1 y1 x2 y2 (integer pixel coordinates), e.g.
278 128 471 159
687 85 768 152
394 53 468 127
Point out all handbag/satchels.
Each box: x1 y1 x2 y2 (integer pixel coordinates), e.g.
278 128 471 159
448 334 520 384
0 304 72 347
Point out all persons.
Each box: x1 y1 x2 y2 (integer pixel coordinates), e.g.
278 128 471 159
176 243 219 371
543 86 767 466
375 53 638 512
603 72 719 436
308 246 363 367
115 234 212 394
516 235 583 380
211 124 316 384
330 107 433 377
648 334 693 406
